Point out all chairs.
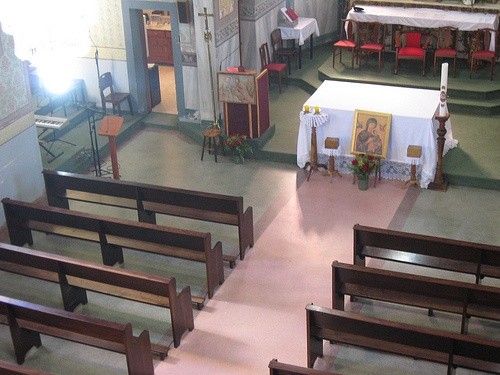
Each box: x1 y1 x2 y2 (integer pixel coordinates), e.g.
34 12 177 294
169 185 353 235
469 28 499 81
270 28 298 75
357 21 388 73
332 18 360 68
259 42 289 93
394 28 431 76
99 71 134 118
433 26 458 77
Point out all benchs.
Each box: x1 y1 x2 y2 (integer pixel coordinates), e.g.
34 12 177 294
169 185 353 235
0 168 253 375
267 221 500 375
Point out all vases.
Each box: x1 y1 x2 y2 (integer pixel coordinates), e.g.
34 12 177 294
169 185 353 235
235 154 241 163
358 177 368 191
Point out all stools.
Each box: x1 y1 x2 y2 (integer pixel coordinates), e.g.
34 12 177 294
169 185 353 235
200 129 225 163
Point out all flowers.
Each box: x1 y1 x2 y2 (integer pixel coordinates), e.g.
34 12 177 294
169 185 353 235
223 132 253 163
348 153 383 179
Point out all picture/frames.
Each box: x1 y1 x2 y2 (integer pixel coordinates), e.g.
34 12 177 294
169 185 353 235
349 108 393 158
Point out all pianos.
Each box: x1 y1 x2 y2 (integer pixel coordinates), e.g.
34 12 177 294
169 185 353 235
33 115 70 163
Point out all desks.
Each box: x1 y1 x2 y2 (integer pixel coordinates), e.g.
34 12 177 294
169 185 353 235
343 4 499 58
295 80 460 188
277 17 320 69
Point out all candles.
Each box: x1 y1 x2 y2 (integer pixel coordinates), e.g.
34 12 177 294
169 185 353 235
305 105 318 112
439 62 449 116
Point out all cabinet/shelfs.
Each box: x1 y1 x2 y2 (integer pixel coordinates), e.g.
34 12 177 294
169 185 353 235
146 29 174 64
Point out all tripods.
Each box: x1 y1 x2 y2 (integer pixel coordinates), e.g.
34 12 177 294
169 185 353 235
75 103 112 176
